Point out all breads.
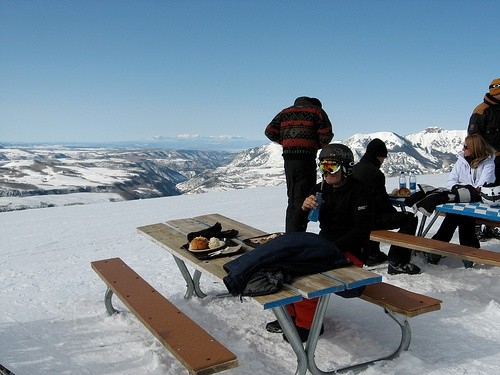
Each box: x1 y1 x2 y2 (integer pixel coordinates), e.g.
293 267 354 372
392 188 398 195
208 237 220 248
396 187 411 196
190 236 208 250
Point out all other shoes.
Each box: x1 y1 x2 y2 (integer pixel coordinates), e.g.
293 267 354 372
368 250 389 266
427 254 440 265
280 323 325 344
464 260 474 269
387 260 423 275
266 320 285 333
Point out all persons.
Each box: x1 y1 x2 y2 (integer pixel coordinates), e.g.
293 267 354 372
351 137 421 275
420 134 496 269
266 142 370 344
467 78 500 241
264 96 334 232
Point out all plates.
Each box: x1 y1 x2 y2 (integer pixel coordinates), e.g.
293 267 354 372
188 240 225 252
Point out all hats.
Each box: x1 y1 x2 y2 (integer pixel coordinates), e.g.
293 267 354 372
366 137 388 158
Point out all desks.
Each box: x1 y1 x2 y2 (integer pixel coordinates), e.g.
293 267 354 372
388 194 500 256
105 214 411 375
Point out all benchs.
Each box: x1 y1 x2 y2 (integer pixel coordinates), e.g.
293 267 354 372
91 258 239 375
359 283 440 318
368 230 500 266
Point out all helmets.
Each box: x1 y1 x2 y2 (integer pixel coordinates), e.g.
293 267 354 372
480 180 500 207
318 143 355 185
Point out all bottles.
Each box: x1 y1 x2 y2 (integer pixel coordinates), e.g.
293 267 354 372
307 192 324 222
409 171 416 194
399 172 406 189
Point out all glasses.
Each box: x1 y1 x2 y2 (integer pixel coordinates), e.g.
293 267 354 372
488 83 500 90
463 144 470 151
318 160 341 175
